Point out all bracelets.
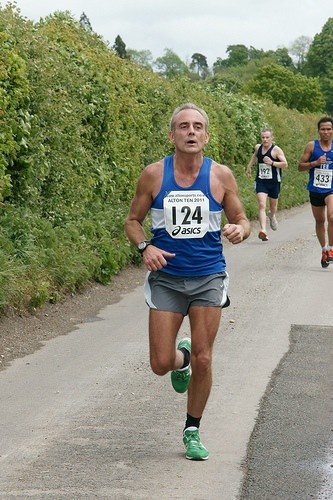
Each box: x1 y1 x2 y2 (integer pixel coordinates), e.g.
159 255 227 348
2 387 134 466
271 160 274 166
310 162 314 168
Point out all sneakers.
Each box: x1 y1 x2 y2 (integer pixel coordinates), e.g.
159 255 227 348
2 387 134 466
183 426 209 460
326 248 333 264
321 250 329 268
267 210 278 231
171 337 191 393
259 230 268 241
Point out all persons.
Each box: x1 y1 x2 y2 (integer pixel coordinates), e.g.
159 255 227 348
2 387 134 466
124 103 252 460
298 117 333 268
246 129 288 241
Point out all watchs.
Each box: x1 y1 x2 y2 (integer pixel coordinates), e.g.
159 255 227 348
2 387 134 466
138 241 153 257
